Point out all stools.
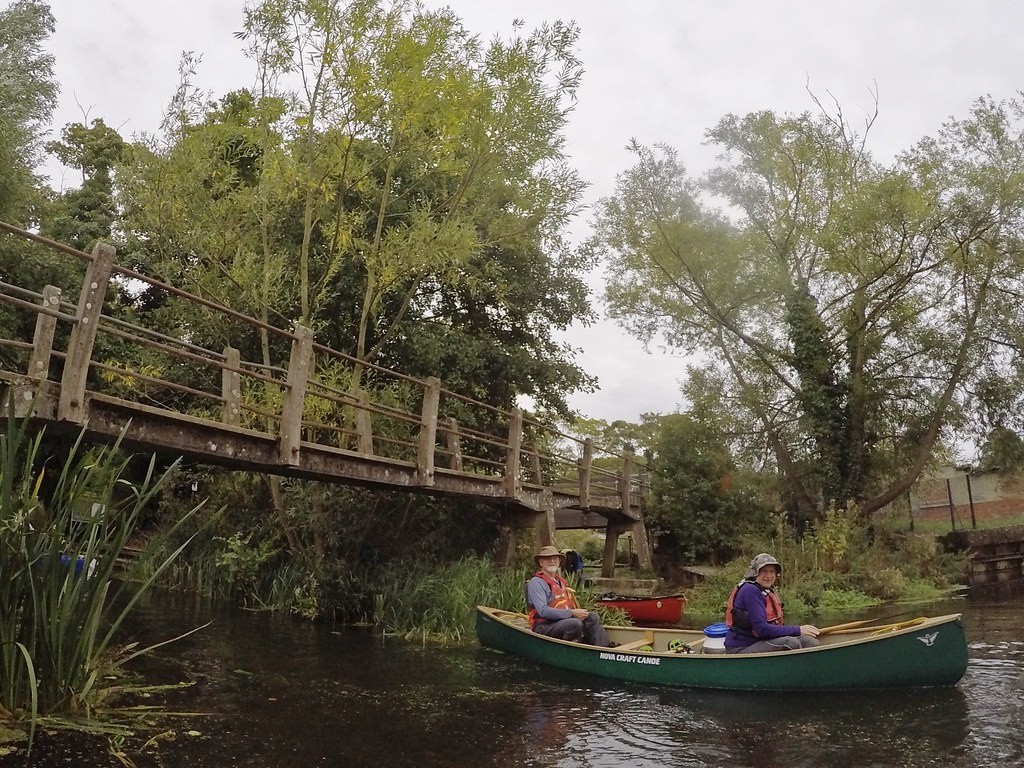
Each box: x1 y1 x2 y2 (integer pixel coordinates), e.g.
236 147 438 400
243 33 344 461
612 639 654 651
665 637 707 653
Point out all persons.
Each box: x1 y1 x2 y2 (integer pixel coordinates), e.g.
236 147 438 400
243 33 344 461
724 553 820 654
524 546 608 647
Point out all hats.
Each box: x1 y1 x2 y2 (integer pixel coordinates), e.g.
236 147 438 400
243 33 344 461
744 553 782 578
534 545 566 568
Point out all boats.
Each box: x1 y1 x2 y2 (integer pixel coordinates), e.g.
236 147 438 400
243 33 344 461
474 607 970 694
59 554 87 578
594 593 685 624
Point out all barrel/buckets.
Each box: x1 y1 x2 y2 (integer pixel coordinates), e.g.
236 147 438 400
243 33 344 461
703 622 730 654
